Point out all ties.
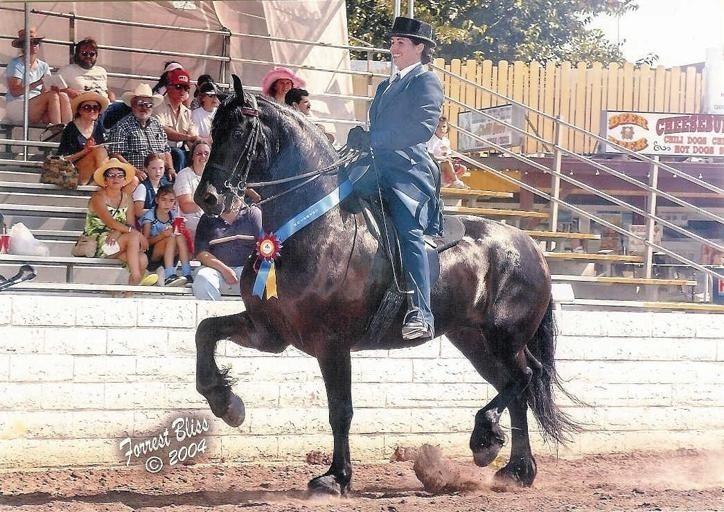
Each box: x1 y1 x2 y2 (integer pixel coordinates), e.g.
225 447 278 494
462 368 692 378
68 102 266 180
383 75 401 95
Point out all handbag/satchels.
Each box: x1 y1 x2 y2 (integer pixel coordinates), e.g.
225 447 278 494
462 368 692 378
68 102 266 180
71 233 99 258
40 154 80 192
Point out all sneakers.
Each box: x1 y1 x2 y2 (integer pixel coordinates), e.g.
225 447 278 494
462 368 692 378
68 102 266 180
450 180 468 189
164 274 187 287
183 275 192 288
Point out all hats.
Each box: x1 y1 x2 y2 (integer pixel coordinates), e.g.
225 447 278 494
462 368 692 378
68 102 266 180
11 30 45 48
387 17 437 49
262 67 305 96
167 70 190 86
120 83 163 108
159 63 185 77
93 158 135 189
70 92 108 114
200 82 218 95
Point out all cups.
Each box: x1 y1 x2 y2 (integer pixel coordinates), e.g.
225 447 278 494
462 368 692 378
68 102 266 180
172 217 185 235
0 235 11 254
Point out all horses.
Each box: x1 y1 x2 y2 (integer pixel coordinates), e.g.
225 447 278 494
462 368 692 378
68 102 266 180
194 71 597 497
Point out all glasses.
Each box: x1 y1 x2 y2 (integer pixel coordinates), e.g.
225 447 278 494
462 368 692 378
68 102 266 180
104 173 124 178
208 95 217 97
176 85 187 91
79 51 95 57
136 100 153 108
31 40 39 46
80 104 100 112
195 152 209 156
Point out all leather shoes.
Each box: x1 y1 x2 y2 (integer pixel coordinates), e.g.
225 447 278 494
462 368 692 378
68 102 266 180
401 315 431 340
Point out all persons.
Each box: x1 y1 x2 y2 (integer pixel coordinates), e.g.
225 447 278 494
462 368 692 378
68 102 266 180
426 116 467 188
3 27 335 299
345 14 445 341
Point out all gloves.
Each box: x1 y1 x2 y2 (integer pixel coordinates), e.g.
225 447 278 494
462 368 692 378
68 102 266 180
347 127 371 152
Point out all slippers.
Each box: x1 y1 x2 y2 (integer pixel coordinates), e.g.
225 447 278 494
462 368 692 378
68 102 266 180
138 272 158 286
